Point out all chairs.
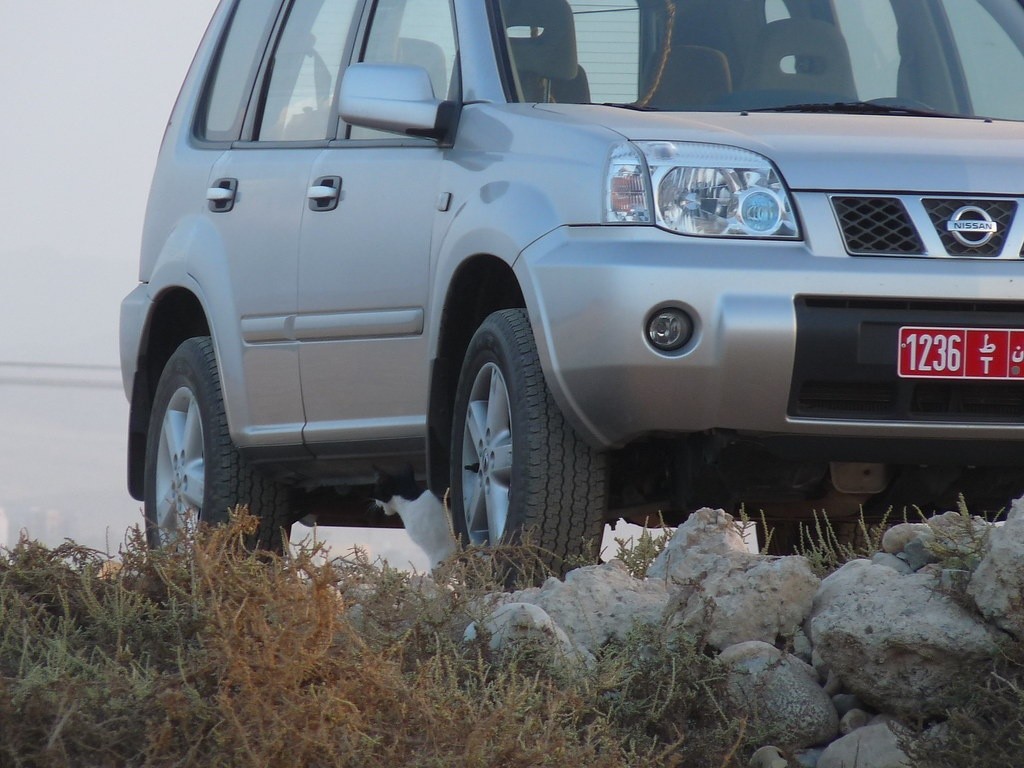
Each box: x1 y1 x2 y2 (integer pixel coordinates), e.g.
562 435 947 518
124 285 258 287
447 0 591 105
732 17 860 104
641 44 733 112
394 37 448 99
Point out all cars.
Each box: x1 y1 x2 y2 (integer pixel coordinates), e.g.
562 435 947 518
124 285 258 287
117 0 1024 594
